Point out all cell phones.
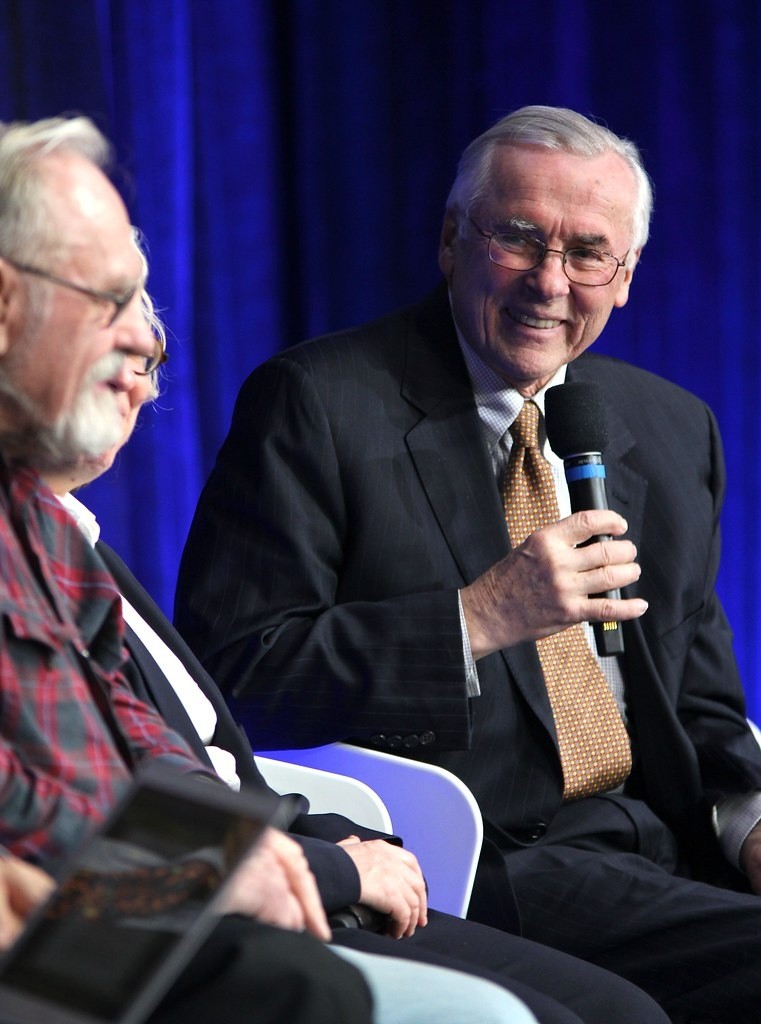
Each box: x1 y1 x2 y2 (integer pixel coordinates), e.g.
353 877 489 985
1 768 292 1024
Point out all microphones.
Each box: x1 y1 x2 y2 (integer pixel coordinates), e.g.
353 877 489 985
543 380 623 658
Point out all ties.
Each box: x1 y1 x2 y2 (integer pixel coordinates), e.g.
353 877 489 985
501 402 633 805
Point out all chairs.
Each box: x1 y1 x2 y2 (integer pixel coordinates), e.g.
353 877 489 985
254 740 485 919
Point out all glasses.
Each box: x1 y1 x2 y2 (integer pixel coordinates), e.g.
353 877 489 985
133 335 169 376
469 217 630 286
0 251 137 328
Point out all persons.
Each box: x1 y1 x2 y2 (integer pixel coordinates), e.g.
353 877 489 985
172 105 761 1024
0 117 669 1024
